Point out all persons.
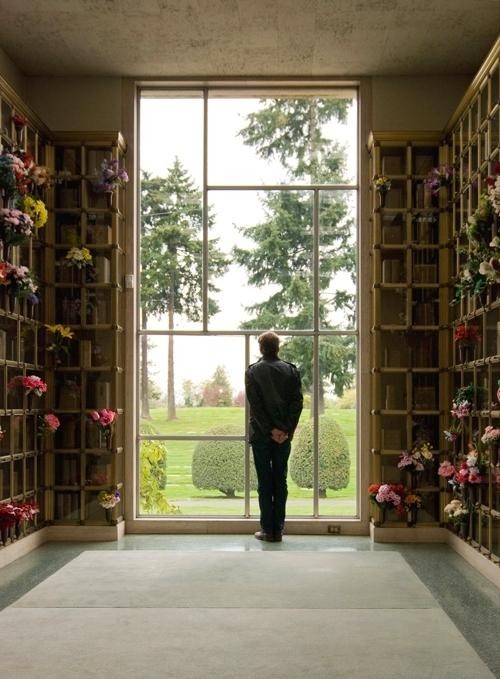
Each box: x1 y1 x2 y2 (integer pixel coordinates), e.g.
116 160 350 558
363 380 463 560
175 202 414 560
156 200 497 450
245 332 303 541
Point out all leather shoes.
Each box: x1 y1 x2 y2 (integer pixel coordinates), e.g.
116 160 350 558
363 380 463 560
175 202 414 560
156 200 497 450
254 530 282 541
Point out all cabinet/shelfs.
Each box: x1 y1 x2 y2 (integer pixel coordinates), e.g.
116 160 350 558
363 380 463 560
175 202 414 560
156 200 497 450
366 34 500 588
0 76 124 567
